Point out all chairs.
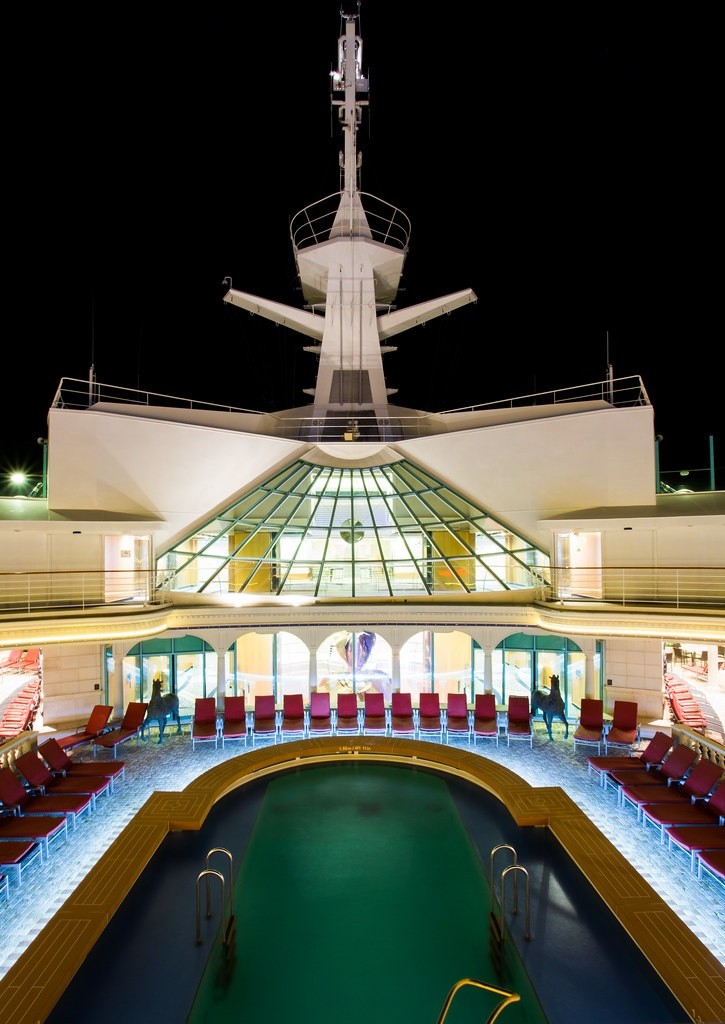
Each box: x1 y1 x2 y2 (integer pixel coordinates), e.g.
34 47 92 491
0 646 125 900
472 694 500 747
307 692 334 738
506 695 535 750
389 693 416 740
361 692 388 736
250 696 278 747
416 693 444 744
589 669 725 886
571 699 606 758
217 697 249 750
279 694 307 743
190 697 219 752
444 693 471 746
91 701 148 761
334 693 361 736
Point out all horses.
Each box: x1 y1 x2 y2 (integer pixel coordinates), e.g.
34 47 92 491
530 675 568 742
141 679 181 744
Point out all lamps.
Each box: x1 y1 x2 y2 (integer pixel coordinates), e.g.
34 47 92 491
378 420 389 425
313 420 323 425
